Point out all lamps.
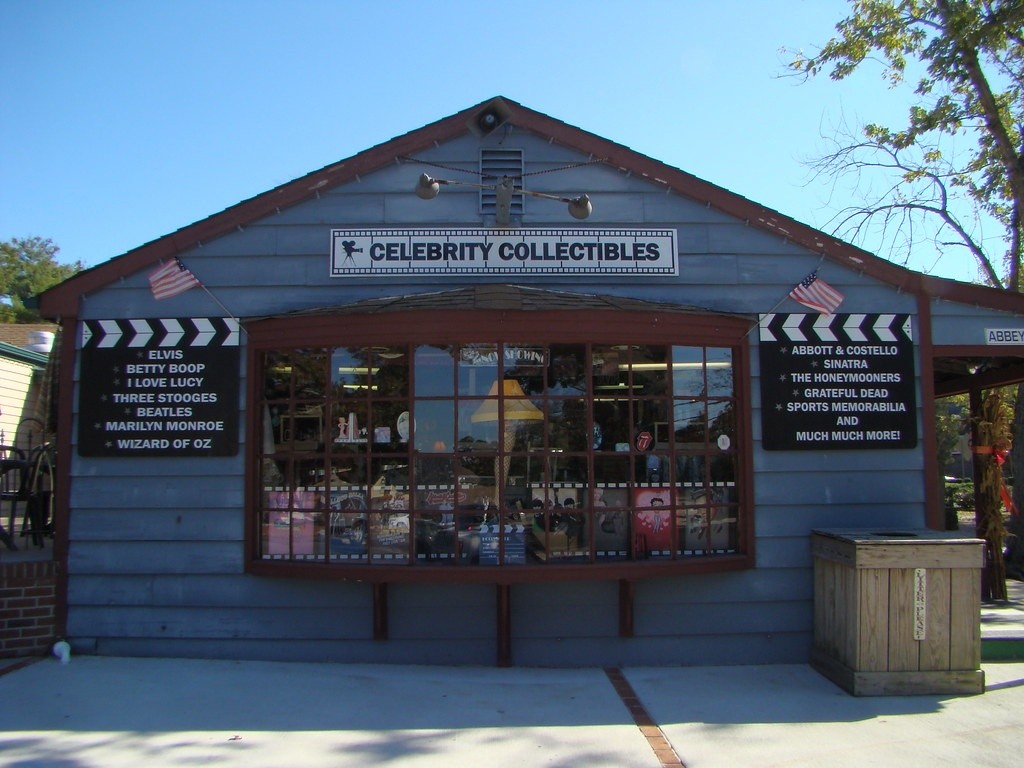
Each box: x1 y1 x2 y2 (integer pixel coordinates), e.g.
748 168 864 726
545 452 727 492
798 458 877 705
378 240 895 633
512 186 593 219
471 379 544 507
416 173 496 200
967 358 991 374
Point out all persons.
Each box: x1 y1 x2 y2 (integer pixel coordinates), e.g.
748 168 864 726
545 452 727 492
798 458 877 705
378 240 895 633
684 488 730 550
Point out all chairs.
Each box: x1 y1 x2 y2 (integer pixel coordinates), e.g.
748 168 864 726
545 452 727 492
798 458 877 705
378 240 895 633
0 447 51 545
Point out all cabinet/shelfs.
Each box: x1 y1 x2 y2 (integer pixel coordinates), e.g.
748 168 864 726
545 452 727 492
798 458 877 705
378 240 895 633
280 414 323 445
647 422 706 443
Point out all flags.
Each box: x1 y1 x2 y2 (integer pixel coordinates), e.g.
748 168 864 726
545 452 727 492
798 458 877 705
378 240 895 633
789 272 846 318
146 256 201 301
